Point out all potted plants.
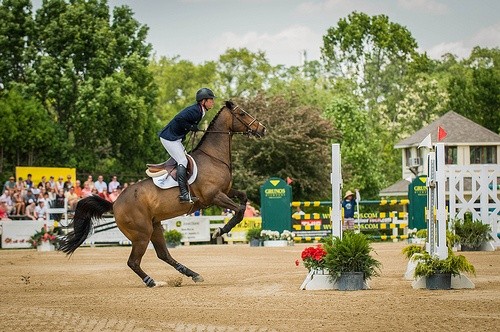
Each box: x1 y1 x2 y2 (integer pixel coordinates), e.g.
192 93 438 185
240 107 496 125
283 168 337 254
323 228 384 291
246 229 259 246
255 229 265 246
165 229 183 248
398 218 494 289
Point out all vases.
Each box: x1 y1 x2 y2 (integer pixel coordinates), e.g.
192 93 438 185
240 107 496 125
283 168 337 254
37 240 56 251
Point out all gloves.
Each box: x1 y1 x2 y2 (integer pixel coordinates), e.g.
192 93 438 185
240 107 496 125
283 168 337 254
188 124 199 132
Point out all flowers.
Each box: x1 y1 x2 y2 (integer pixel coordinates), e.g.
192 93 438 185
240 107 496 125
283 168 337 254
295 244 328 270
29 225 65 248
260 229 292 241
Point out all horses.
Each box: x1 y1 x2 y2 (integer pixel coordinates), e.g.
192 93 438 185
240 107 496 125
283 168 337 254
56 98 268 288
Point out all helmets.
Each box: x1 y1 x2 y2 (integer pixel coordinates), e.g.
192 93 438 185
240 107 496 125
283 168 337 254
196 88 217 101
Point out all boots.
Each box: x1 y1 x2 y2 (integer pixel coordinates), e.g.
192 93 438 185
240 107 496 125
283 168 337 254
177 164 200 203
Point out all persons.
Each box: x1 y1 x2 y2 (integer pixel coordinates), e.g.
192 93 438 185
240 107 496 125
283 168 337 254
108 176 127 202
342 189 361 230
294 207 306 214
159 88 217 203
244 201 258 216
64 175 82 209
222 208 235 216
191 209 203 216
93 175 110 202
81 176 95 198
0 174 64 221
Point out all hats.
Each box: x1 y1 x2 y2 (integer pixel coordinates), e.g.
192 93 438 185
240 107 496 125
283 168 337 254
9 177 14 179
28 199 33 203
343 190 354 199
